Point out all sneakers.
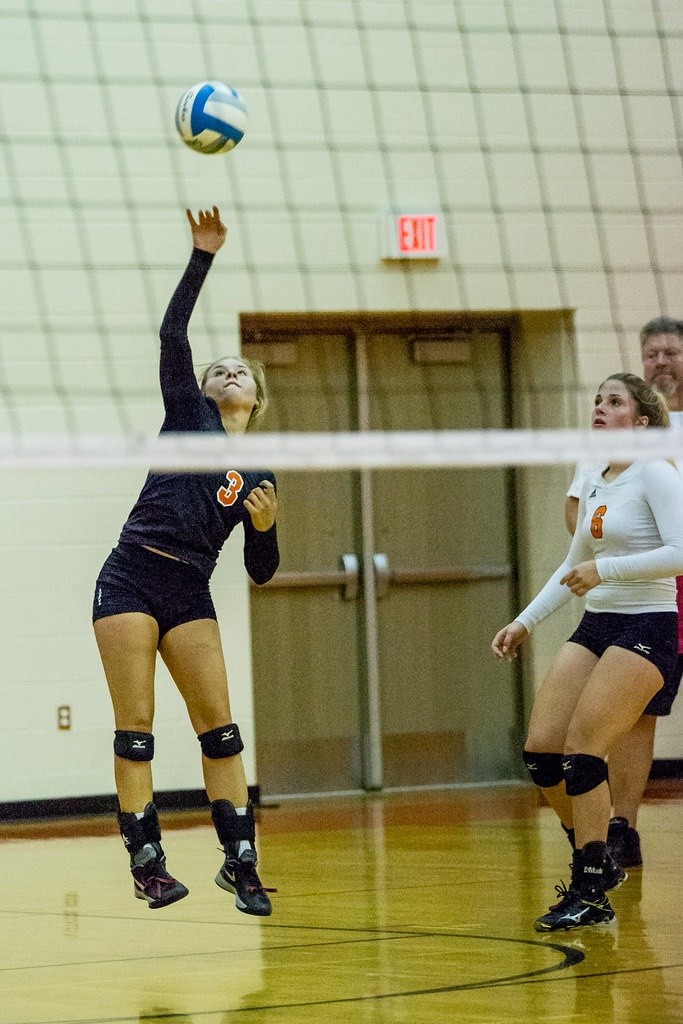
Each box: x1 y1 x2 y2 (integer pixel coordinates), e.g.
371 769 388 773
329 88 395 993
214 846 278 917
601 853 629 896
605 815 643 871
129 842 189 909
532 878 615 932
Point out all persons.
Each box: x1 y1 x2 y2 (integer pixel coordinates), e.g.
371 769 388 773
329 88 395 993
564 317 683 869
489 374 683 932
93 205 281 916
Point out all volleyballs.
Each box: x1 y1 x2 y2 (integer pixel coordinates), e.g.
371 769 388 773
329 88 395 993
175 81 248 154
